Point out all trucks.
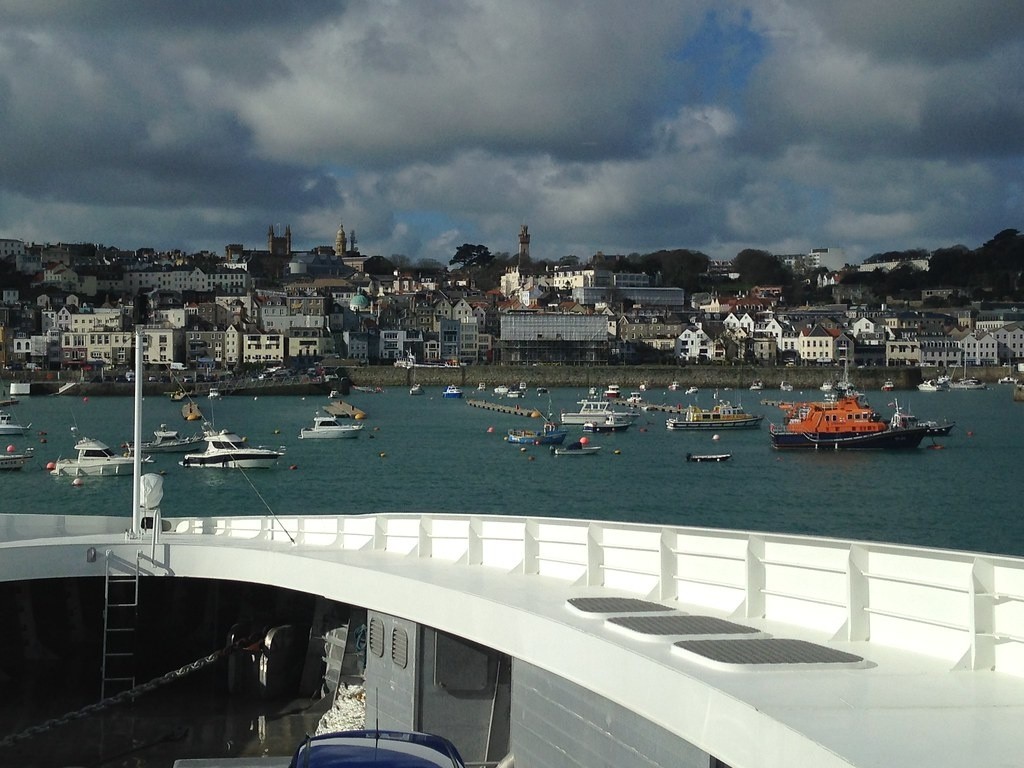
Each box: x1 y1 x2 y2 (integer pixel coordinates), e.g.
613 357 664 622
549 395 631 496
170 362 188 371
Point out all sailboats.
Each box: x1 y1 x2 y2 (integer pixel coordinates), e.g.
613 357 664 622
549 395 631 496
998 358 1020 385
916 336 988 391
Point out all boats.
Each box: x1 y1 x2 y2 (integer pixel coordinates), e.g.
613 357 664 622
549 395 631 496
442 383 464 399
559 377 640 426
410 382 426 395
50 408 154 478
553 441 601 455
664 387 767 432
880 397 956 437
182 401 203 422
686 452 733 462
582 412 632 433
0 444 35 471
0 408 33 436
328 389 343 399
178 395 286 470
880 378 895 393
125 422 206 454
206 387 222 400
297 403 367 440
478 344 855 404
507 406 569 445
768 343 929 450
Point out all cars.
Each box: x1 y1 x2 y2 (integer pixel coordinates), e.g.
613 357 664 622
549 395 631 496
289 730 465 768
103 365 317 383
